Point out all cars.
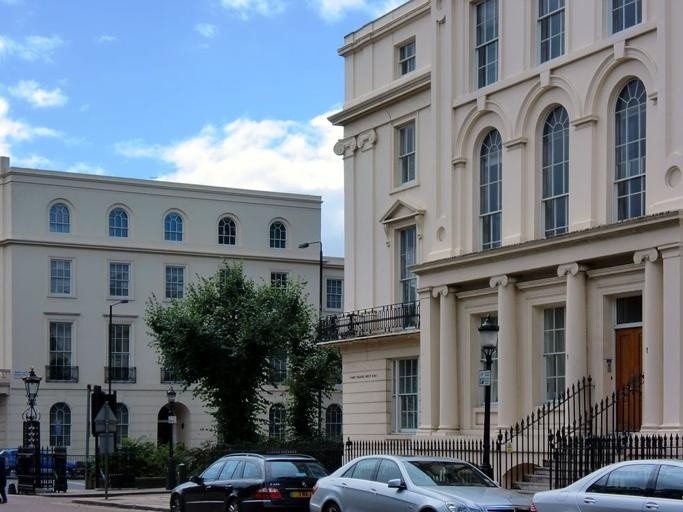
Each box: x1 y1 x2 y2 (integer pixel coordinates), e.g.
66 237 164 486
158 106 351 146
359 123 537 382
532 458 683 511
309 454 537 512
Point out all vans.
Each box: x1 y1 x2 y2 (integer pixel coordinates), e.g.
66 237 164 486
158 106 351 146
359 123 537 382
0 448 78 479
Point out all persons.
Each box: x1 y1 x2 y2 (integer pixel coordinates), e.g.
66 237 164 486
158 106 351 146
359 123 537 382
0 456 7 504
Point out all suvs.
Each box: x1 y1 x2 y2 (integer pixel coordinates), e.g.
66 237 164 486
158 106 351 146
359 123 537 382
169 452 331 512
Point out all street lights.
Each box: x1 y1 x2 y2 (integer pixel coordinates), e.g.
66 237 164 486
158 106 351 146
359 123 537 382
298 241 322 437
109 300 128 394
478 312 499 466
166 386 176 456
21 365 41 422
